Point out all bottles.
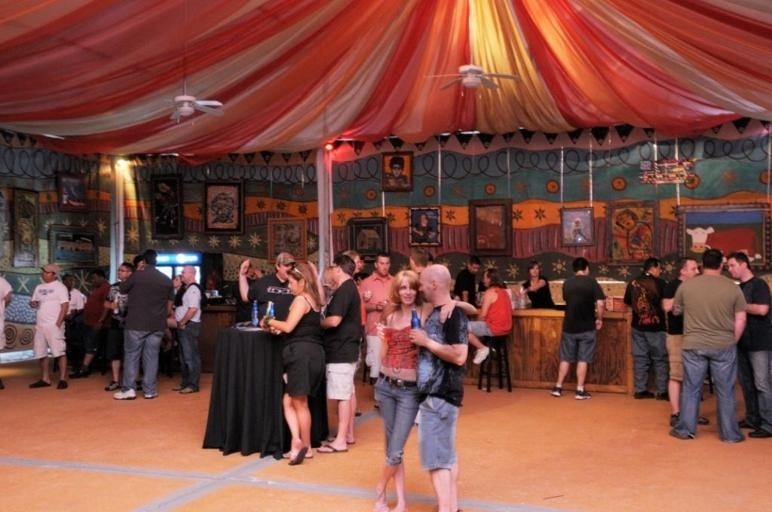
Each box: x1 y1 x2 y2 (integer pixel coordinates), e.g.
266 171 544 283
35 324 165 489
518 287 526 309
463 291 468 302
411 309 421 329
252 300 276 326
114 294 120 314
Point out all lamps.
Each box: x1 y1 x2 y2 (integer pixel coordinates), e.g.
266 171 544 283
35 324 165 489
176 102 195 117
462 73 483 87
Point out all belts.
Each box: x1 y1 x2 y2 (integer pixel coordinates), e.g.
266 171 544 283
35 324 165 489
378 372 417 390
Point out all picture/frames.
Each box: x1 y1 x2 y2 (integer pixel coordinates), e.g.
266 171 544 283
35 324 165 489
55 170 90 213
346 217 389 262
606 200 660 266
468 199 512 256
202 179 246 235
407 206 442 247
267 217 308 264
674 202 772 273
559 207 595 247
148 174 185 240
48 224 99 268
380 151 415 192
11 186 40 269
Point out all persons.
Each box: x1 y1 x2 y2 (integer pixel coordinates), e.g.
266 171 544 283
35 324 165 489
232 248 515 465
384 156 408 187
26 249 207 401
620 250 771 444
549 255 605 401
412 212 433 243
0 276 15 390
154 183 177 207
520 259 555 309
375 263 478 511
572 217 589 242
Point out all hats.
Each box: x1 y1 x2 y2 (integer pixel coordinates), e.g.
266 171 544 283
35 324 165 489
41 264 57 274
277 253 296 265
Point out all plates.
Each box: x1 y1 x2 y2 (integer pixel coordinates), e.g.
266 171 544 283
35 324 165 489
605 295 628 312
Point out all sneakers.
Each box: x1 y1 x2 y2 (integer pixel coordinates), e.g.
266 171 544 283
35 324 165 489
635 390 669 399
551 386 561 396
473 347 490 365
671 412 708 427
172 383 197 394
106 379 159 400
575 391 592 399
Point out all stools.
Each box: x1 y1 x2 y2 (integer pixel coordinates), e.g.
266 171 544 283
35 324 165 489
476 336 513 392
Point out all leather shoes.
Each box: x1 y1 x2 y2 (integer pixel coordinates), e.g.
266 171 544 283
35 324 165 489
737 418 770 437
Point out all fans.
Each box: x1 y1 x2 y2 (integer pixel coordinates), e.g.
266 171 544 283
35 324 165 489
156 95 224 120
422 64 519 91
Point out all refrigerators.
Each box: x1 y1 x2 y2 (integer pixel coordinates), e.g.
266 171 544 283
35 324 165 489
154 252 223 295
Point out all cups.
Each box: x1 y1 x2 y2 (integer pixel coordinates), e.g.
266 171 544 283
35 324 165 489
212 290 218 296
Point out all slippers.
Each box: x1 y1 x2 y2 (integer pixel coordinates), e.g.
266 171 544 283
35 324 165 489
56 379 68 389
283 434 355 465
29 378 53 388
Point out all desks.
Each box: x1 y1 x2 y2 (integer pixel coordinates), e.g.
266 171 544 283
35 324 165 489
200 319 329 465
